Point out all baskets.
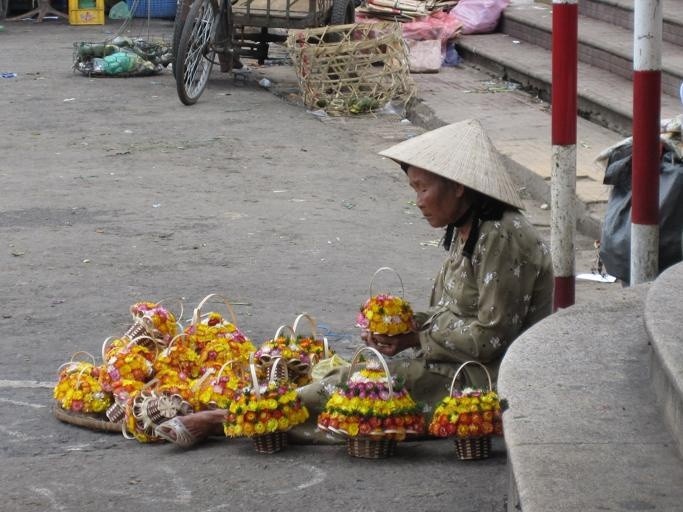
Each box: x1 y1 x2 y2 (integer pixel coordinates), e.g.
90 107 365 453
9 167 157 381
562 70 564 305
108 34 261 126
450 361 495 461
287 24 417 111
55 267 418 459
55 302 418 459
360 264 415 354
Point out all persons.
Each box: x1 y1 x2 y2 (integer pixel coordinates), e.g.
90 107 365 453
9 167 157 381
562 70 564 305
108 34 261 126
155 161 555 448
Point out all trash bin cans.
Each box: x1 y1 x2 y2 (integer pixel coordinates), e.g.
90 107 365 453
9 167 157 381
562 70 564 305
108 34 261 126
282 16 417 117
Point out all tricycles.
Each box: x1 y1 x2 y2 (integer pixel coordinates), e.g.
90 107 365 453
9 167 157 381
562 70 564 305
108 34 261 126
171 0 355 106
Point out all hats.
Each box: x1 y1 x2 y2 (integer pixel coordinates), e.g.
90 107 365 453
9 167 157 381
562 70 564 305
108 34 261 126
379 120 525 209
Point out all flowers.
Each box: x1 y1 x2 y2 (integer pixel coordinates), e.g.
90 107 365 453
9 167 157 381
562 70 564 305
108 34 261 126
257 334 335 378
219 383 309 441
352 296 418 336
428 392 501 437
316 359 427 435
51 303 257 434
